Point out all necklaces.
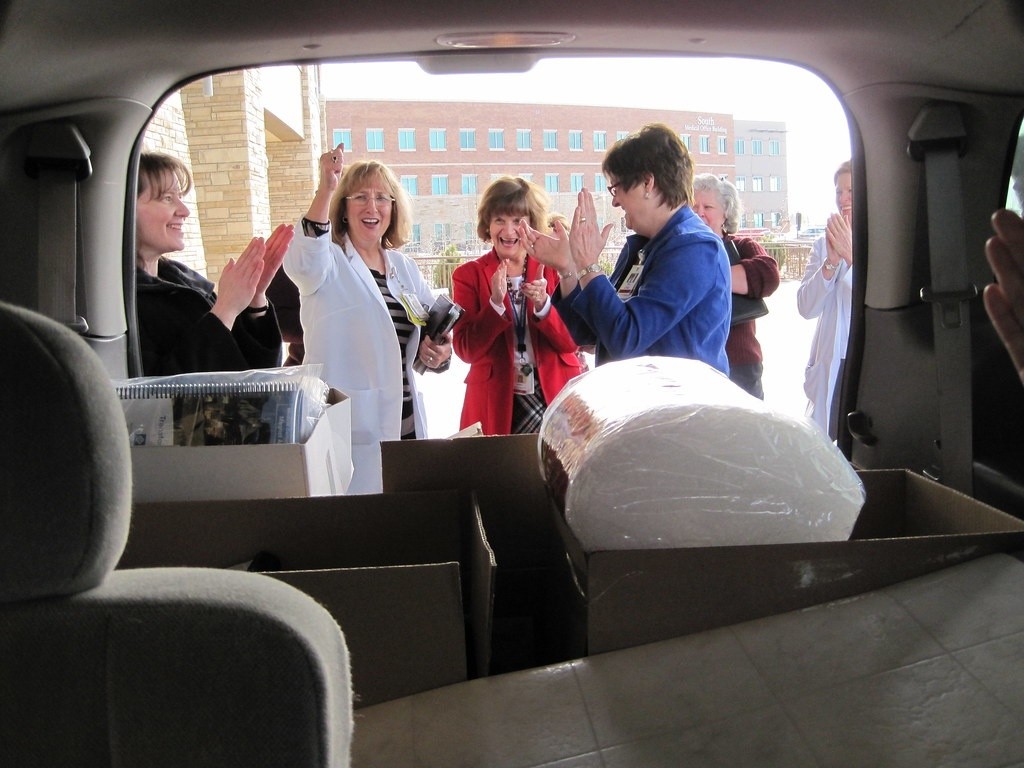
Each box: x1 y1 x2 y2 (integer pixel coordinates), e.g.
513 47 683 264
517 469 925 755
506 256 527 305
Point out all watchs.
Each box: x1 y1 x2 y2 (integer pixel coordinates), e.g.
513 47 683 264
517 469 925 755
824 258 839 271
576 263 602 280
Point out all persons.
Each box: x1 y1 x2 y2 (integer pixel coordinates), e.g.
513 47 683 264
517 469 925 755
450 177 595 435
796 161 852 441
283 142 454 495
545 216 596 374
137 153 295 378
687 173 780 400
518 124 732 380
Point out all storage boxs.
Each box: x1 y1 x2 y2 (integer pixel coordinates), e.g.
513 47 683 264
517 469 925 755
103 357 1023 709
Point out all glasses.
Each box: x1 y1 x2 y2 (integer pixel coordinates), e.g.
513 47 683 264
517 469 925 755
345 194 396 206
607 183 621 197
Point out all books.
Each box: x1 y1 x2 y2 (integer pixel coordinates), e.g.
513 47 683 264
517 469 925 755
115 380 304 446
413 293 465 376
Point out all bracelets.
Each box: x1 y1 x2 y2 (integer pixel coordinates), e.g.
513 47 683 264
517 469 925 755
250 300 268 313
558 271 572 279
438 349 452 368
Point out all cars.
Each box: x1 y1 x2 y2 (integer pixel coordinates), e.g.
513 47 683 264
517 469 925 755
736 228 768 241
800 227 826 237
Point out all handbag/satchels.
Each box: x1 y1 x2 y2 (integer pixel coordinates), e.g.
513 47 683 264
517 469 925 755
722 240 769 327
131 386 355 503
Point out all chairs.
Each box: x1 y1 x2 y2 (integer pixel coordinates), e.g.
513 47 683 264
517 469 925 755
0 302 357 768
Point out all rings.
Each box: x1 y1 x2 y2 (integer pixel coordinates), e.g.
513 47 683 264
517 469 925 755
428 357 432 362
530 244 533 247
579 218 586 223
332 156 337 163
